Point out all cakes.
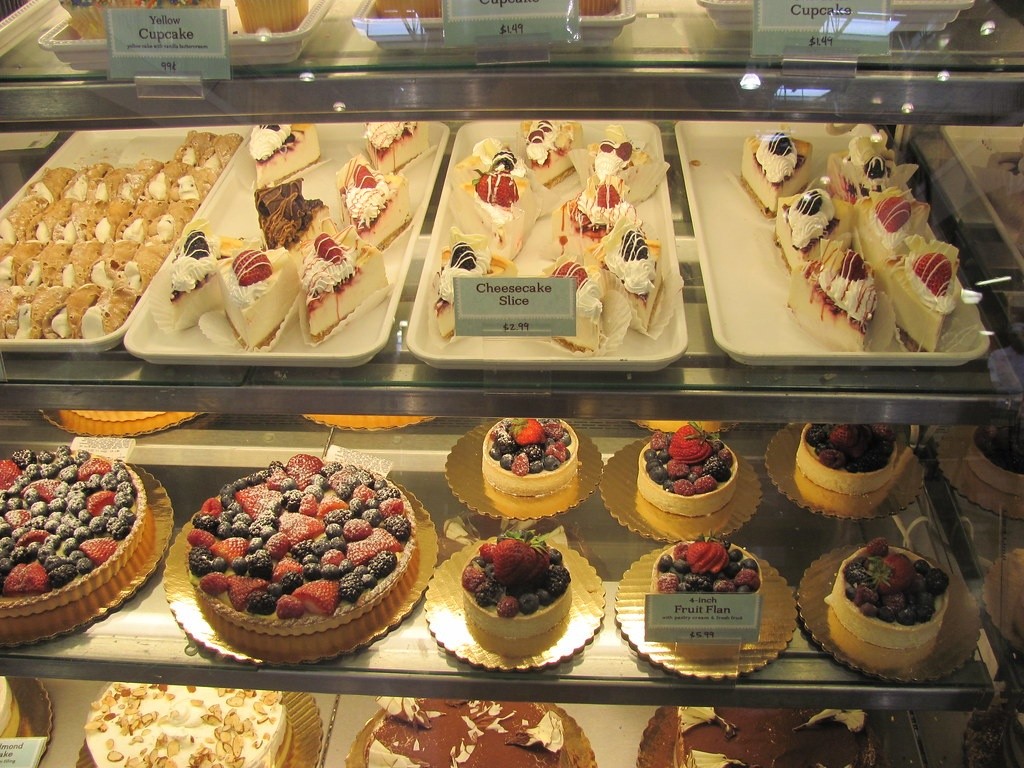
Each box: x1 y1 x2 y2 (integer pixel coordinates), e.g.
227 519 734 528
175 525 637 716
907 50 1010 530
635 704 877 768
302 414 430 429
462 530 572 639
637 421 739 517
0 675 15 738
650 528 763 593
84 681 288 768
0 444 147 621
482 417 579 497
966 421 1023 495
633 420 730 432
365 695 573 768
58 410 200 435
186 453 419 636
794 421 898 495
823 538 951 650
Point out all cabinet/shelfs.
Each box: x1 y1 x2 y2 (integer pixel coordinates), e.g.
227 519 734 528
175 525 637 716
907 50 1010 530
1 1 1022 768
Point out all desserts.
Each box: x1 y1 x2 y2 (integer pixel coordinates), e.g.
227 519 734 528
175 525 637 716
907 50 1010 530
741 131 960 353
0 130 244 340
57 0 308 39
170 121 436 353
431 121 666 356
